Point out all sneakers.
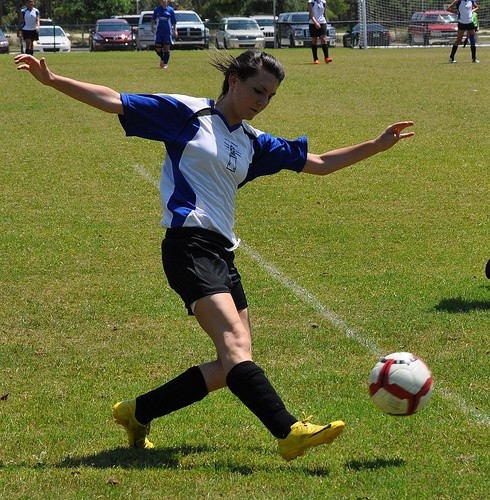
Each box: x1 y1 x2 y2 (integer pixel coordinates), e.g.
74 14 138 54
277 412 345 462
113 398 153 449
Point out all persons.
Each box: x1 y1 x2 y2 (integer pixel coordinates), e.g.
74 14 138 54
308 0 332 64
462 7 478 48
13 43 415 461
446 0 480 63
151 0 178 69
17 0 40 56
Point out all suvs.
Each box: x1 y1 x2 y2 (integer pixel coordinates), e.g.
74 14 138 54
169 10 210 49
276 12 337 48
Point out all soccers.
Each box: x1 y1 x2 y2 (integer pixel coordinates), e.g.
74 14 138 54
368 351 433 417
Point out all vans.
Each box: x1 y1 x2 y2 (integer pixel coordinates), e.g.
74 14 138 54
407 11 467 46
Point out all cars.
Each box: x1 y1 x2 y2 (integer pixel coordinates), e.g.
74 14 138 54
110 14 141 40
137 11 157 51
0 29 11 53
248 15 279 43
88 18 138 52
343 22 391 47
39 18 55 26
215 16 265 49
18 25 71 53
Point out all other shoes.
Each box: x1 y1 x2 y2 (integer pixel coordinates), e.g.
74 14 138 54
162 64 167 68
325 58 332 63
449 57 456 62
314 60 319 64
160 60 164 67
472 60 479 63
